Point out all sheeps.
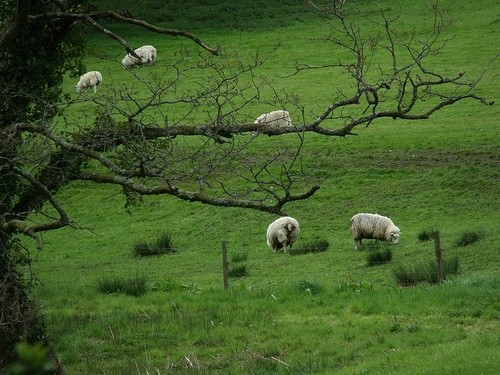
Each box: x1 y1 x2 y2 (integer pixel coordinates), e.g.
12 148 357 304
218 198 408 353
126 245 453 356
251 110 293 136
266 216 300 254
351 213 402 250
121 45 156 70
75 71 102 93
